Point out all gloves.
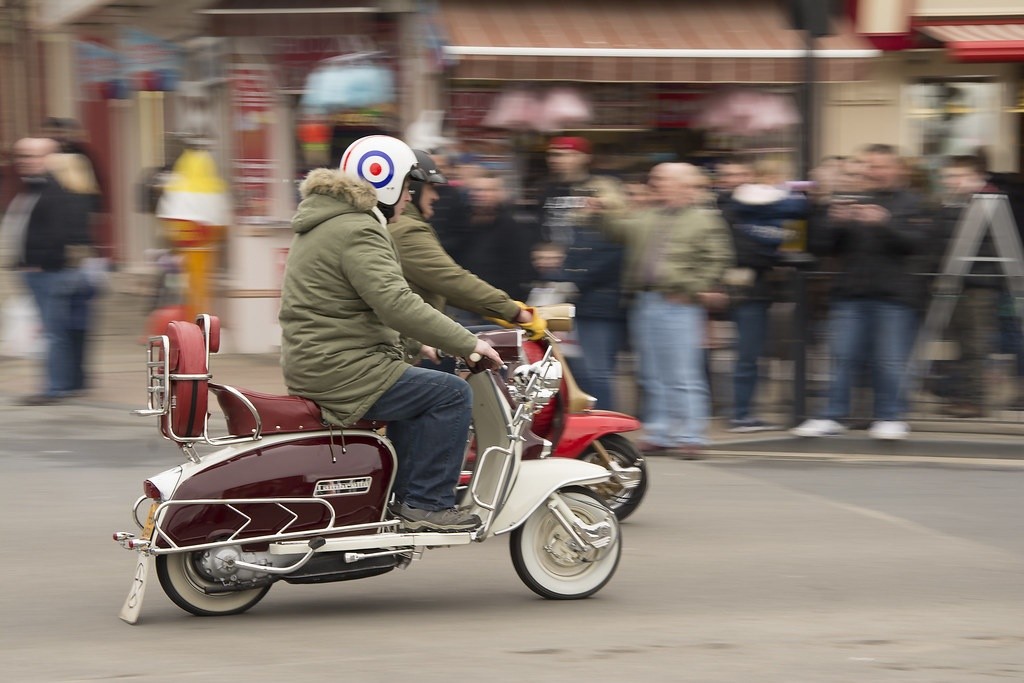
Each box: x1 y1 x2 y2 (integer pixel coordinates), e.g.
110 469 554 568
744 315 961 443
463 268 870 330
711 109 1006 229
516 306 548 341
484 297 528 328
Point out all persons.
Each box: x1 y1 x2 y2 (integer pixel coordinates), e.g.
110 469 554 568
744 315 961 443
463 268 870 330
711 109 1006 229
1 109 107 408
158 121 233 323
275 136 505 532
366 115 1024 448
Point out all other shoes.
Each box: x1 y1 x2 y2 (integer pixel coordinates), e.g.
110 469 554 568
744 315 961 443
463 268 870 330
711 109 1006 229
674 439 708 459
723 417 786 433
635 437 676 456
788 418 850 437
868 420 910 440
20 386 74 407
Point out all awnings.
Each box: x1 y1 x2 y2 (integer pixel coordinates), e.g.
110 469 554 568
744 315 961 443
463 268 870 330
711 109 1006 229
417 6 875 86
911 19 1024 63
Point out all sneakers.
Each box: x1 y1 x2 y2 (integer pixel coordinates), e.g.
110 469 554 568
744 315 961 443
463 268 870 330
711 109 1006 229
383 496 404 532
401 502 482 533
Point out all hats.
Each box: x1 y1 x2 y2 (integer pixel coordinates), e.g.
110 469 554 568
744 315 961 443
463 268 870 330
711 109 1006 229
545 135 594 154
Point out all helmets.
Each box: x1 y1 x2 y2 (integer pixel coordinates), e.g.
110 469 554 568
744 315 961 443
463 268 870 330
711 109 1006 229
408 149 449 185
339 134 419 207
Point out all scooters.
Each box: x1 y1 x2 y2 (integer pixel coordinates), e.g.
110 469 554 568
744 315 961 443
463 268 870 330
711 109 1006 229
112 300 650 625
